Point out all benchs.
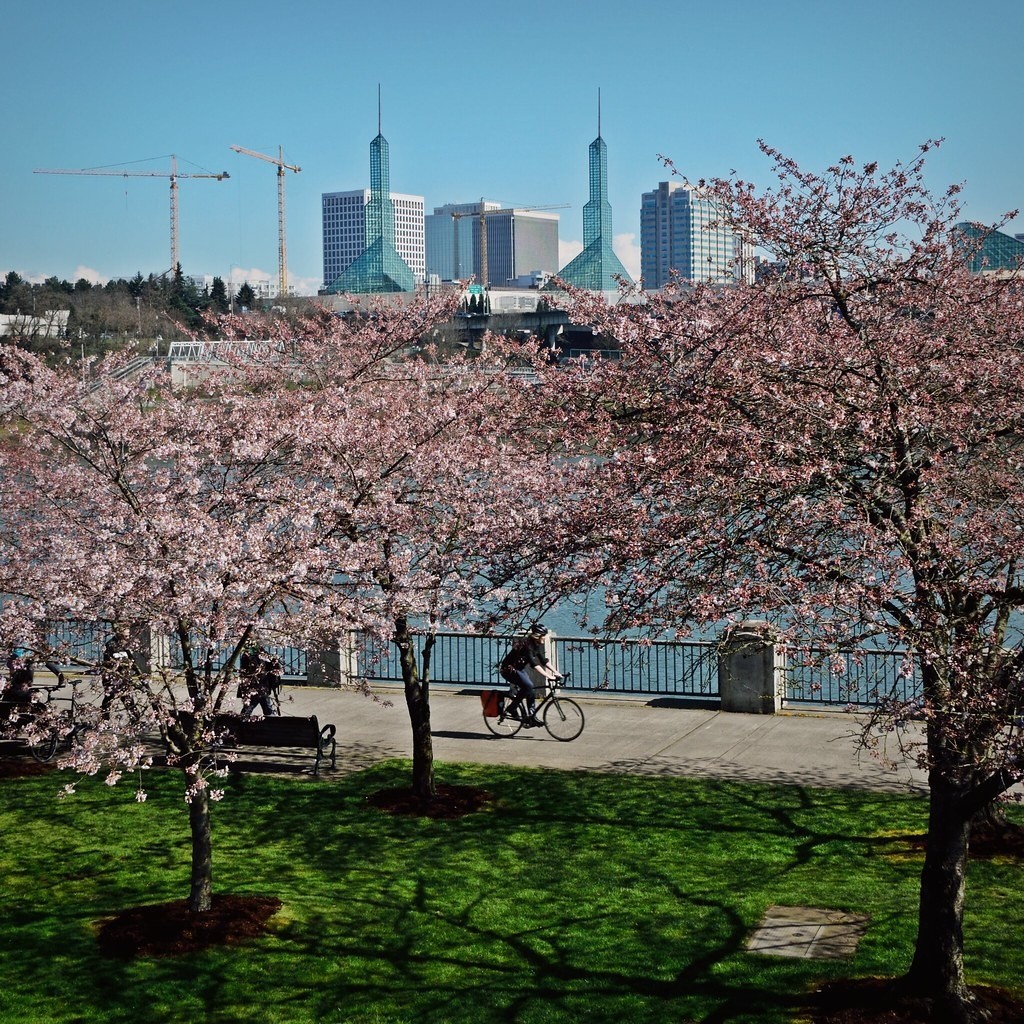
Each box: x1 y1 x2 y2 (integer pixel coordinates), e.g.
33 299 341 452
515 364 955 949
166 710 339 776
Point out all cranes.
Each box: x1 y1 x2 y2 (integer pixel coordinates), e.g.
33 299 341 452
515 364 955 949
229 145 303 299
33 155 230 278
451 197 571 291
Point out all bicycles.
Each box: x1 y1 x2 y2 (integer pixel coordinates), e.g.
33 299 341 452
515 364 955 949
27 680 101 762
482 672 585 742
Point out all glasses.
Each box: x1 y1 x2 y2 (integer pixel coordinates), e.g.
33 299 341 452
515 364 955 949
541 634 545 636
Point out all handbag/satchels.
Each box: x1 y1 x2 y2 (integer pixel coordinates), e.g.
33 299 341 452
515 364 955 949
481 690 499 717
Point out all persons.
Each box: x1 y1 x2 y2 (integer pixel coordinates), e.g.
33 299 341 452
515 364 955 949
236 649 286 716
500 624 563 728
102 626 139 719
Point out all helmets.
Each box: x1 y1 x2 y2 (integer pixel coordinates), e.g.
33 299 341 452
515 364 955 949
532 624 548 634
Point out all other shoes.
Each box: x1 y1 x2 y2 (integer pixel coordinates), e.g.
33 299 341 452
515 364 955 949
505 705 519 720
528 716 544 726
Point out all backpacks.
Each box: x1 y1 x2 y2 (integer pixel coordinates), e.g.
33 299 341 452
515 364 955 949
243 649 281 690
